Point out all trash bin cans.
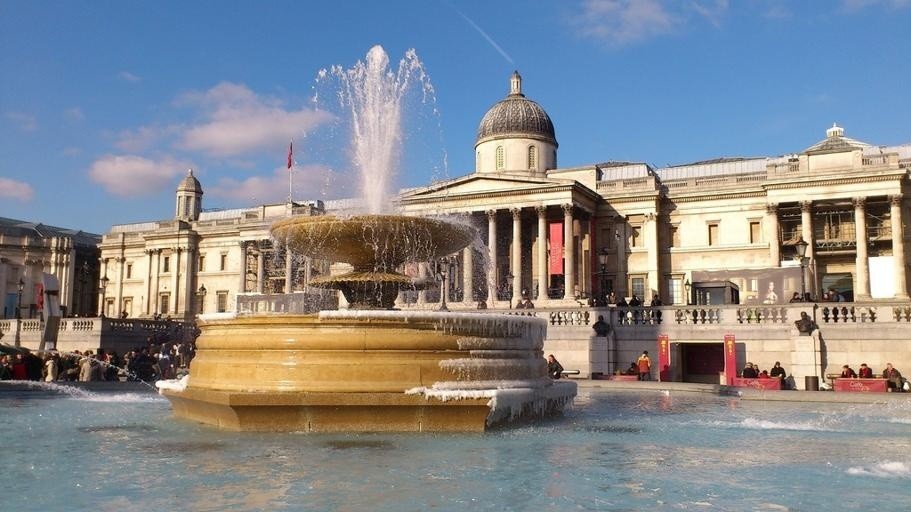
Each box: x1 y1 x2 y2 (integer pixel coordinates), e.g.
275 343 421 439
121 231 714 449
805 376 818 391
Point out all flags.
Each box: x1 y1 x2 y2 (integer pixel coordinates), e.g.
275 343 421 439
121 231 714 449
286 140 292 169
548 223 563 275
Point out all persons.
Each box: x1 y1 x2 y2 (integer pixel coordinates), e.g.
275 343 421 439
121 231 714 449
743 362 786 391
402 282 528 301
0 316 195 382
841 365 857 378
625 351 653 382
859 363 872 378
883 363 903 392
515 298 536 316
789 288 840 303
548 354 564 379
592 292 663 324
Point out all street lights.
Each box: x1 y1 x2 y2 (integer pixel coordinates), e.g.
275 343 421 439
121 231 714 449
506 271 516 309
78 261 90 317
597 247 609 307
199 284 207 313
16 278 24 351
683 279 692 305
99 275 110 318
793 235 810 302
439 256 449 312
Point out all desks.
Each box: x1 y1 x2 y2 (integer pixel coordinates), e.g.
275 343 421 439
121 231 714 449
597 373 641 383
729 376 783 391
833 377 890 393
